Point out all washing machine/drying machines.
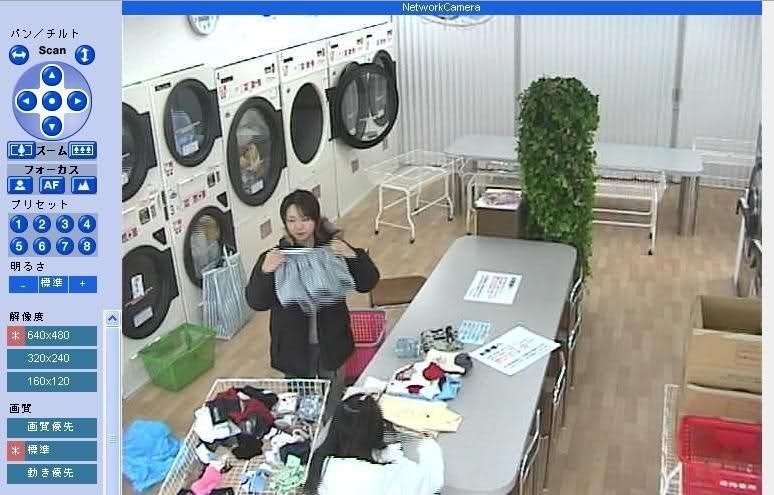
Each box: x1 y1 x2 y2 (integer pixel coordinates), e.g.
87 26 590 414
120 20 244 398
210 22 402 282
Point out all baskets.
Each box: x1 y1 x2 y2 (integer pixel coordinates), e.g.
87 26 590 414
676 416 762 495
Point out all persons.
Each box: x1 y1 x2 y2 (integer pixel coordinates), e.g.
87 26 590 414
245 190 379 427
302 393 445 495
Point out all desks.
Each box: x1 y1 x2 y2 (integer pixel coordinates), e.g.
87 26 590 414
313 235 578 495
464 170 667 255
444 133 702 235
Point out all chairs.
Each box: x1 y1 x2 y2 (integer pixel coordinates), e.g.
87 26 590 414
518 266 584 495
365 248 428 308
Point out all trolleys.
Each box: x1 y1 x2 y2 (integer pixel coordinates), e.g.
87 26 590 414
157 375 333 495
458 155 668 255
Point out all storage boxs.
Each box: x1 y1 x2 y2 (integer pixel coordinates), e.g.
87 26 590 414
675 295 762 450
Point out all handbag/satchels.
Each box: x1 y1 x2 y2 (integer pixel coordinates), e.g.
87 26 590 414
202 251 254 340
